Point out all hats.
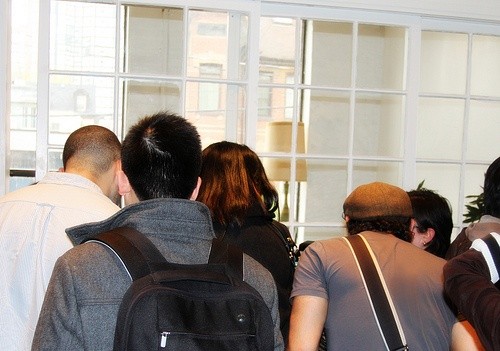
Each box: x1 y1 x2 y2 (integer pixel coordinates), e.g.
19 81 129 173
342 182 413 219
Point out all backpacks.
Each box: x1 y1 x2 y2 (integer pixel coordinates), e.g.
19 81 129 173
80 225 274 351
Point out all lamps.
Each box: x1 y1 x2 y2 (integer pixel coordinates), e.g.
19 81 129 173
256 121 308 222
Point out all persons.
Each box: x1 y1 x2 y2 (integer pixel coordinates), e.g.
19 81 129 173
31 113 285 351
406 189 453 259
196 141 304 351
0 124 122 351
442 157 500 351
289 182 486 351
